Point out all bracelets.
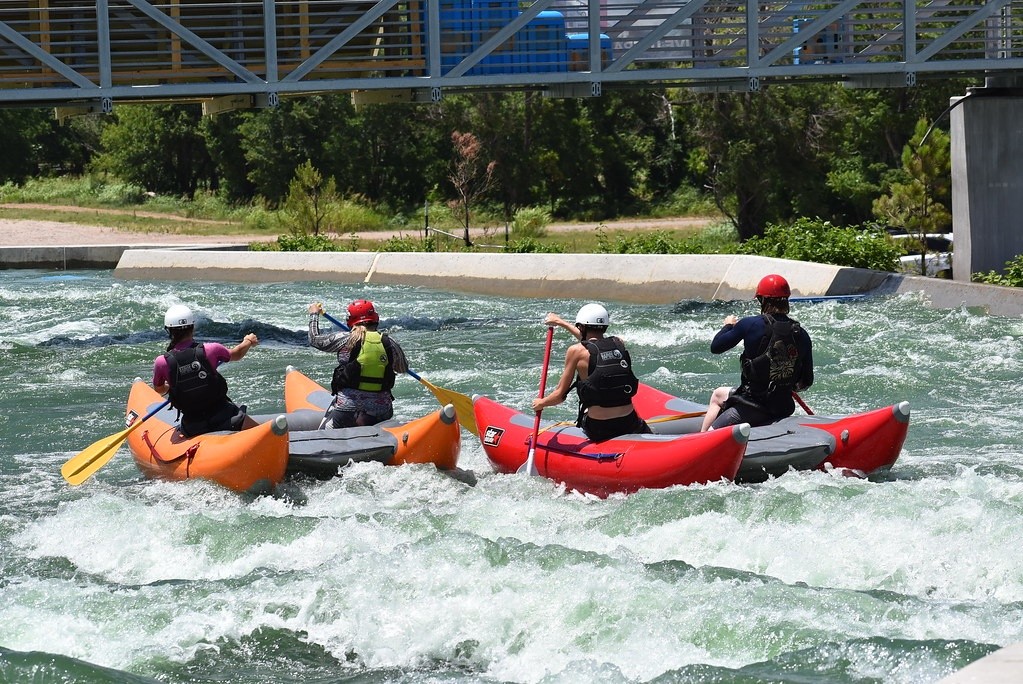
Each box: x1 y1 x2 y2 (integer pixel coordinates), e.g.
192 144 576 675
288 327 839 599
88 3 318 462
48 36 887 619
245 338 253 345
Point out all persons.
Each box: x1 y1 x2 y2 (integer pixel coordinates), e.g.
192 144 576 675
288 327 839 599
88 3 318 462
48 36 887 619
532 303 653 441
308 299 408 429
700 273 814 432
152 304 260 438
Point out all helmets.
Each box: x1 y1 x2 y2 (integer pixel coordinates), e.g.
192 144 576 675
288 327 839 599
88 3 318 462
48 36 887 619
756 275 791 299
345 300 379 326
575 303 609 326
165 305 194 327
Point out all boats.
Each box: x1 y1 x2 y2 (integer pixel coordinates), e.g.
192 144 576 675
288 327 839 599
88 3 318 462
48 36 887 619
470 381 912 504
124 367 461 497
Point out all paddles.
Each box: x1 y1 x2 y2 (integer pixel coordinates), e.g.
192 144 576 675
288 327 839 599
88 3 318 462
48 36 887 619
316 302 480 437
516 322 558 478
60 360 224 486
791 390 815 415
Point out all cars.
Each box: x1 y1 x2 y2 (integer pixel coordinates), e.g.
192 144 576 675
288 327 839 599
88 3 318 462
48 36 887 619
852 230 953 277
